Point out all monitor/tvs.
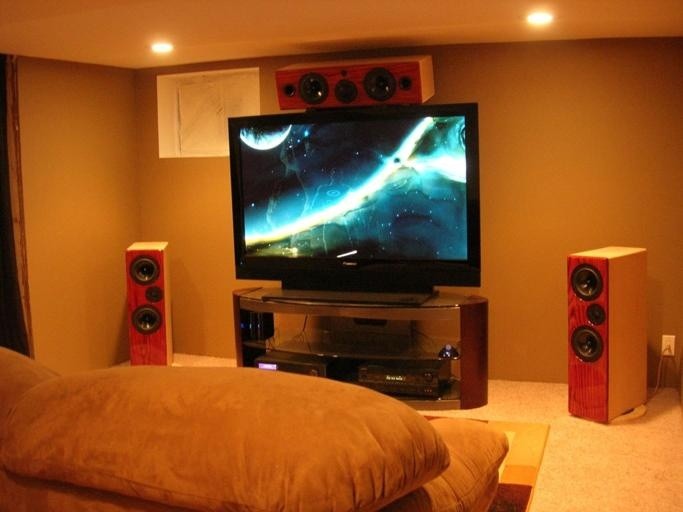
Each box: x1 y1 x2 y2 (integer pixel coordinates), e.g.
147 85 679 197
228 102 481 292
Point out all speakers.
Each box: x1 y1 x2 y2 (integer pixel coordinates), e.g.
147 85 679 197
567 246 648 423
126 241 173 367
274 54 435 110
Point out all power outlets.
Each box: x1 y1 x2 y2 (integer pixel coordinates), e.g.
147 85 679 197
661 335 675 357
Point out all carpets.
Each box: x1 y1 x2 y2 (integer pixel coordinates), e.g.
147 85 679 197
421 413 552 512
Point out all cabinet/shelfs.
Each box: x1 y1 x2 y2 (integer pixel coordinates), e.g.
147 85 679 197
231 285 488 408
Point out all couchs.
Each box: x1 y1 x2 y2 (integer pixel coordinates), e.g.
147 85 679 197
0 409 509 512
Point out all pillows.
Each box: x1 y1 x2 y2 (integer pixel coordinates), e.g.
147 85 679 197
0 364 452 512
0 347 64 418
